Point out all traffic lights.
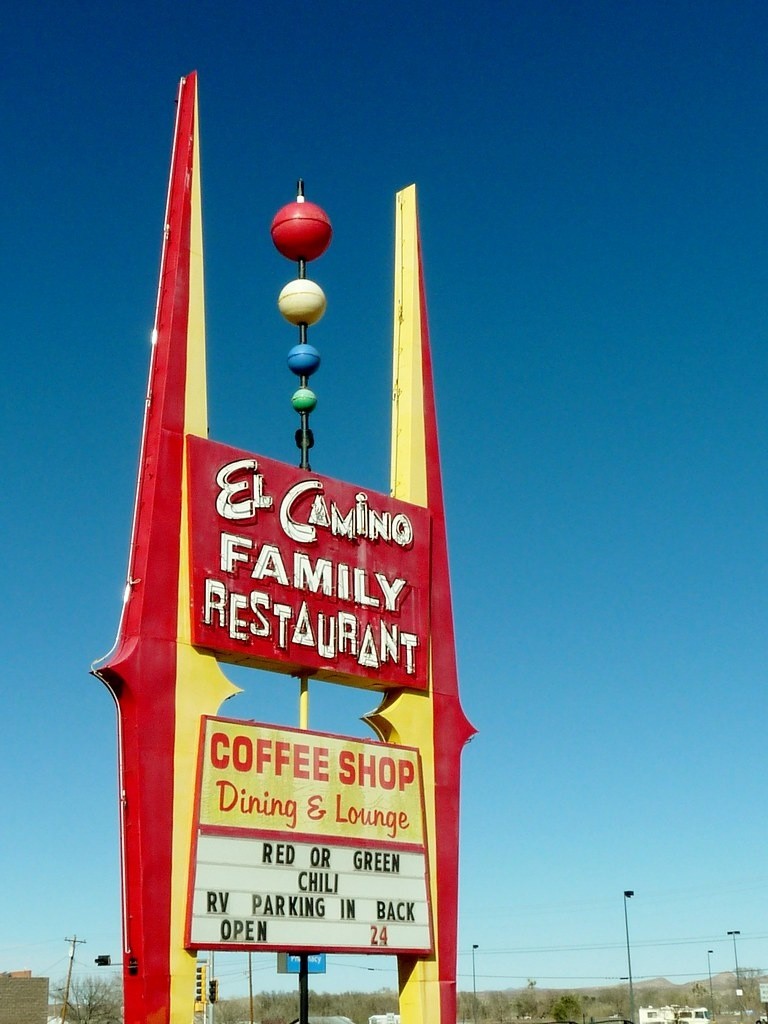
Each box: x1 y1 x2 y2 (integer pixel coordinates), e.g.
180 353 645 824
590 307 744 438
208 981 219 1003
196 966 206 1002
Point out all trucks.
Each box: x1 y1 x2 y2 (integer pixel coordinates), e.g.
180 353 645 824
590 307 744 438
638 1006 710 1024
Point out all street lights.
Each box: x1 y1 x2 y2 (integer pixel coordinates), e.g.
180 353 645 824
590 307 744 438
623 890 637 1024
727 931 743 1022
707 950 715 1021
472 944 479 1024
